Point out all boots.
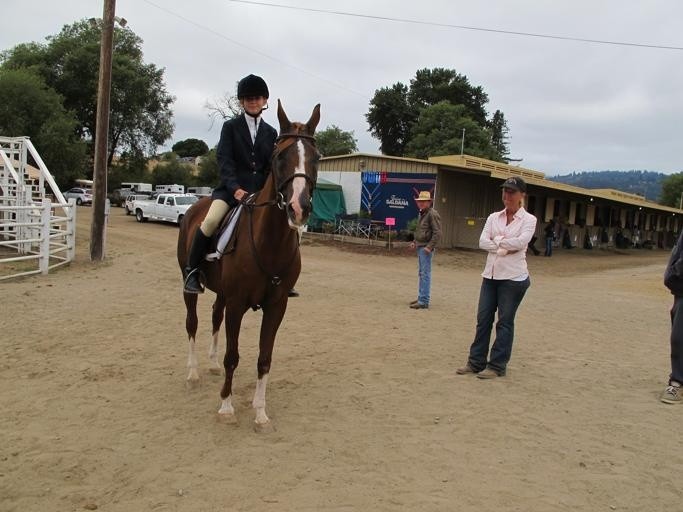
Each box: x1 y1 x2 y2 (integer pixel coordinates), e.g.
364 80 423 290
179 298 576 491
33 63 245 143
183 225 212 294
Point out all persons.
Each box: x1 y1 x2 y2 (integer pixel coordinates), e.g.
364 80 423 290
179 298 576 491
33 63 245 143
455 177 538 381
529 218 683 256
183 73 301 298
660 229 683 405
407 190 443 310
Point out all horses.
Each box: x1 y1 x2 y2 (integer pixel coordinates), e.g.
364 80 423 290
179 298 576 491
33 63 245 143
175 98 321 436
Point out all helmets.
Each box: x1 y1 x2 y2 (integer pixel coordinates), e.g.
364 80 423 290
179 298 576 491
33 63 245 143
237 75 269 100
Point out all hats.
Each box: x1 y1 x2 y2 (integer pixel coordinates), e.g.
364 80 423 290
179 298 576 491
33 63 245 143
499 176 526 191
414 191 434 202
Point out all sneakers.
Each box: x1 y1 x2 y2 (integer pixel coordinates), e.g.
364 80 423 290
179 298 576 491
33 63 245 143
660 379 683 404
476 367 507 379
455 363 482 375
409 300 429 309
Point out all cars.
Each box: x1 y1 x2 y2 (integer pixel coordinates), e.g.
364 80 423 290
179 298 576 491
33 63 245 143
57 177 215 224
